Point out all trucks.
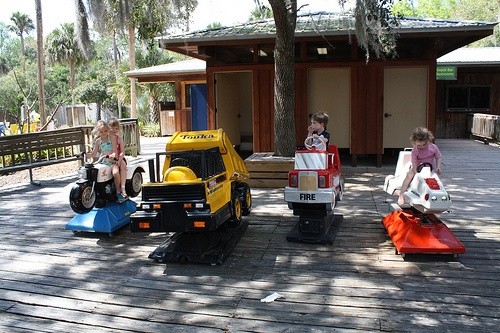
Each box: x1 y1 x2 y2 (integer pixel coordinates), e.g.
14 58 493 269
129 129 251 232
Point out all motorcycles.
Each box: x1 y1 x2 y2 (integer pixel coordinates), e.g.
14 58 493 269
69 152 146 214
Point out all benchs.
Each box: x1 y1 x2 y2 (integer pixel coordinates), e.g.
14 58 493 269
244 152 295 187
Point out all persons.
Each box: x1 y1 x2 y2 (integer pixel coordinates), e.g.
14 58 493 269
397 126 442 206
79 117 129 202
307 111 331 151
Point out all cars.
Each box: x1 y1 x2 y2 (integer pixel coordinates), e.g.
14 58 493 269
284 137 345 210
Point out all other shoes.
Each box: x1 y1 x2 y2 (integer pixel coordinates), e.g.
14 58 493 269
121 191 127 198
117 192 122 201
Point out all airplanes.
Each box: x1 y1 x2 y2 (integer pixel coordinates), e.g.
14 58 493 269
383 148 452 216
